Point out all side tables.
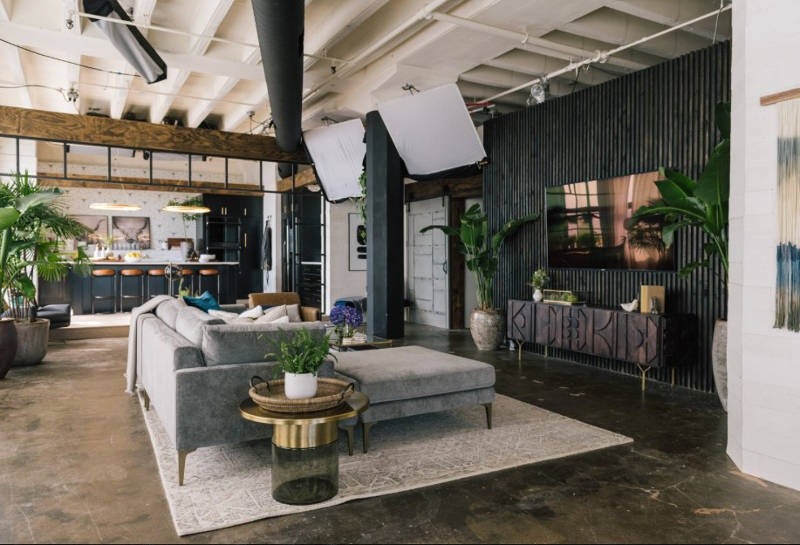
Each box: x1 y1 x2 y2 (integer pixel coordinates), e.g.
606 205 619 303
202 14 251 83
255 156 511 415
240 393 370 505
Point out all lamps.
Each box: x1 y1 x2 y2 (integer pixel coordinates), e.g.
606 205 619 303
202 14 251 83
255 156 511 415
83 0 167 83
89 203 141 211
163 204 212 212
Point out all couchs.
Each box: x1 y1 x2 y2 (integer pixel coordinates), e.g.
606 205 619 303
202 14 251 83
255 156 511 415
247 292 320 324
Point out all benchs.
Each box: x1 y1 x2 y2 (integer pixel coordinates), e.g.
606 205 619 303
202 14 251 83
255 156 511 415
330 345 498 454
128 303 358 485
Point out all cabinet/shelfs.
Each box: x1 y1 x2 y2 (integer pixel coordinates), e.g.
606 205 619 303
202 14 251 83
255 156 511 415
196 194 263 304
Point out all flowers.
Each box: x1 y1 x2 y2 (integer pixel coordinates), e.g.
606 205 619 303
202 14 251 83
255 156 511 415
330 305 360 328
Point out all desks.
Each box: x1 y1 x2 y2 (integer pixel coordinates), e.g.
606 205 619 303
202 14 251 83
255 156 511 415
507 299 676 391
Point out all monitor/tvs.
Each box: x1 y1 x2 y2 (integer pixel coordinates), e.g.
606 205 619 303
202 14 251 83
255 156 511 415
545 169 678 273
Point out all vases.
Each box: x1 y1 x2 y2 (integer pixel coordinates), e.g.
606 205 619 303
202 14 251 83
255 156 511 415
342 324 352 337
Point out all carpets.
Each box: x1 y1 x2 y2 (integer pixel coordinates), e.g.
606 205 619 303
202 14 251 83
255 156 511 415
135 385 635 538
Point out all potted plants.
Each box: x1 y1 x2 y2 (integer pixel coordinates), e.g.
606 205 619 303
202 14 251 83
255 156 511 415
0 169 94 379
419 204 540 349
259 322 339 400
624 98 729 412
528 268 549 302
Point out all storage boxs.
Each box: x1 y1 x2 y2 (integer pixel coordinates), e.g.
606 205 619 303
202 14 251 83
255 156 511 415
543 290 587 307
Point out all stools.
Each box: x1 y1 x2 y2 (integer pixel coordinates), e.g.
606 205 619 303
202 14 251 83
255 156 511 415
91 269 220 313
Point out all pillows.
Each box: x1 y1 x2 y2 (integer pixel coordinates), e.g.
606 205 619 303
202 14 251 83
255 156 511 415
182 290 302 322
